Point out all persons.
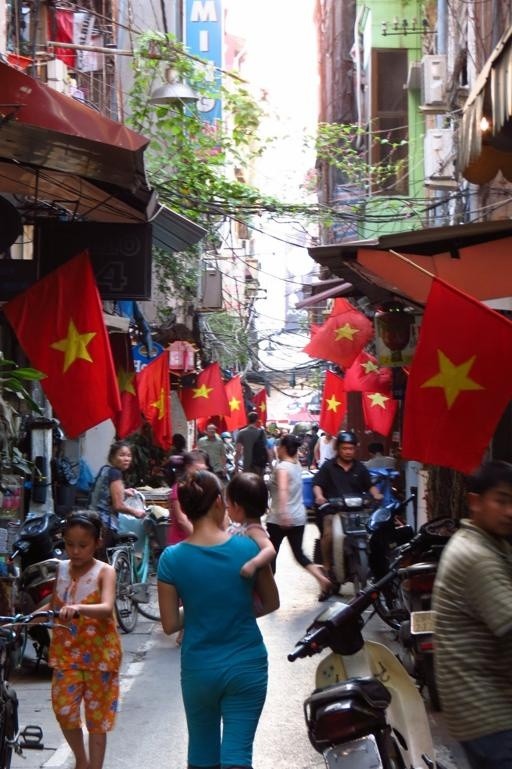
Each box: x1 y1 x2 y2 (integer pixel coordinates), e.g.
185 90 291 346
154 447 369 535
167 450 211 646
266 435 337 602
432 461 512 769
175 472 277 647
306 424 317 469
365 442 396 474
273 428 288 456
236 413 273 474
224 432 237 470
309 431 385 568
313 431 338 465
6 509 122 769
87 442 146 562
156 471 268 769
198 423 228 478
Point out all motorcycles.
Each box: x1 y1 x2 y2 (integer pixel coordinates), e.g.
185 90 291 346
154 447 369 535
312 492 370 586
387 527 439 706
288 569 438 767
355 491 417 631
7 508 72 659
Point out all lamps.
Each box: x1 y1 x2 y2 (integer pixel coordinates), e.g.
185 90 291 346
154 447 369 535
47 42 199 105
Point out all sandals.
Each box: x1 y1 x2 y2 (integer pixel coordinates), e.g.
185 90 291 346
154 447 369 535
318 582 341 603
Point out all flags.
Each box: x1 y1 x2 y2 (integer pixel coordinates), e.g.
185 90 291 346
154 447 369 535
318 371 347 437
196 376 248 435
111 333 144 439
302 297 375 368
178 364 231 419
400 277 512 475
248 389 268 426
308 325 318 336
129 351 172 453
345 326 396 393
361 392 399 438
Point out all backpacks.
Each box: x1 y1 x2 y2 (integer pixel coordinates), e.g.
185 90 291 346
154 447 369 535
251 428 268 469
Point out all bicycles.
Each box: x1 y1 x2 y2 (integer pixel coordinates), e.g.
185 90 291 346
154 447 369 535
1 607 79 769
105 488 172 633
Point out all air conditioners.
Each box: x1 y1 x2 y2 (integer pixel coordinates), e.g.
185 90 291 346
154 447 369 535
419 56 446 107
424 130 455 180
201 269 223 309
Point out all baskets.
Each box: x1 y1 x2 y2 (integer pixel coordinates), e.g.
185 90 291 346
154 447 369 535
340 512 371 537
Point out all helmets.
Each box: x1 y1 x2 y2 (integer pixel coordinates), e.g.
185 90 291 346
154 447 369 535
336 431 359 443
221 432 232 438
